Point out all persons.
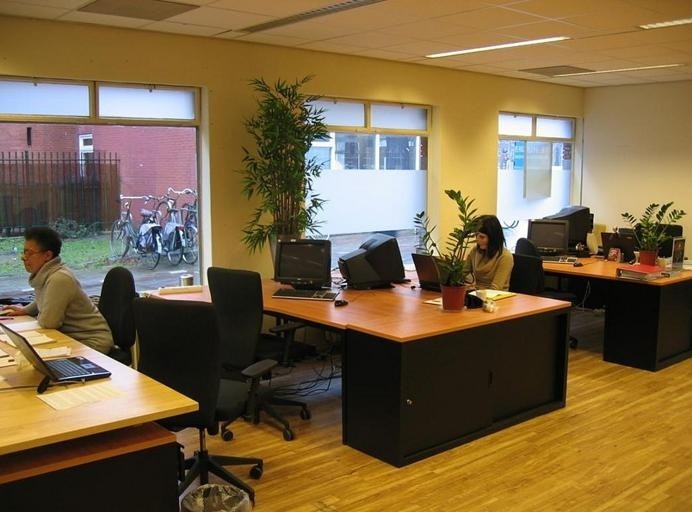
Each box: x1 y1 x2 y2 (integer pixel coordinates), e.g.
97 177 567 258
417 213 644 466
0 227 114 355
464 216 514 292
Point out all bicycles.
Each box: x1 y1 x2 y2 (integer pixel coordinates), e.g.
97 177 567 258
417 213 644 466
106 187 199 268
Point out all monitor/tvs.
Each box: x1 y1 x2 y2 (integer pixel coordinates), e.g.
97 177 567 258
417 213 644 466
338 233 405 289
601 232 642 262
527 219 570 254
274 240 331 290
543 206 590 251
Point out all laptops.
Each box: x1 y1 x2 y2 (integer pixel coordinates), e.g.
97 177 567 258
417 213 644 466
411 253 441 291
0 322 111 382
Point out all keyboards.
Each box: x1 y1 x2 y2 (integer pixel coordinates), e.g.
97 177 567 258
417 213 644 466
542 256 577 264
272 288 339 301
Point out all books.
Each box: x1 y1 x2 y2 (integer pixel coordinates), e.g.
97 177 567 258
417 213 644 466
0 331 56 349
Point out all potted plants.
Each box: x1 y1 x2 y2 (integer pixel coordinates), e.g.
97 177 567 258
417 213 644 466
413 187 488 313
234 68 336 268
622 199 687 268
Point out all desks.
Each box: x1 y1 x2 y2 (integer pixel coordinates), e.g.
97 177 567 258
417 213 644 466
0 302 204 511
152 270 575 470
541 252 692 374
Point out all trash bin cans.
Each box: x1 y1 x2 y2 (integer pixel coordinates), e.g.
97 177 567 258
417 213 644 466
181 483 248 512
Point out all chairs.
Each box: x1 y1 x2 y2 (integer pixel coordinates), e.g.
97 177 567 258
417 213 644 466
514 237 579 348
509 251 544 297
205 265 312 442
96 265 138 368
129 294 265 509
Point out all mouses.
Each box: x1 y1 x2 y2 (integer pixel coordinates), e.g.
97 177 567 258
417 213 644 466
335 299 348 306
573 262 583 266
0 309 15 316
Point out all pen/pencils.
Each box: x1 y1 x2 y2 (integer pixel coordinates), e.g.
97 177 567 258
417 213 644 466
0 317 14 320
